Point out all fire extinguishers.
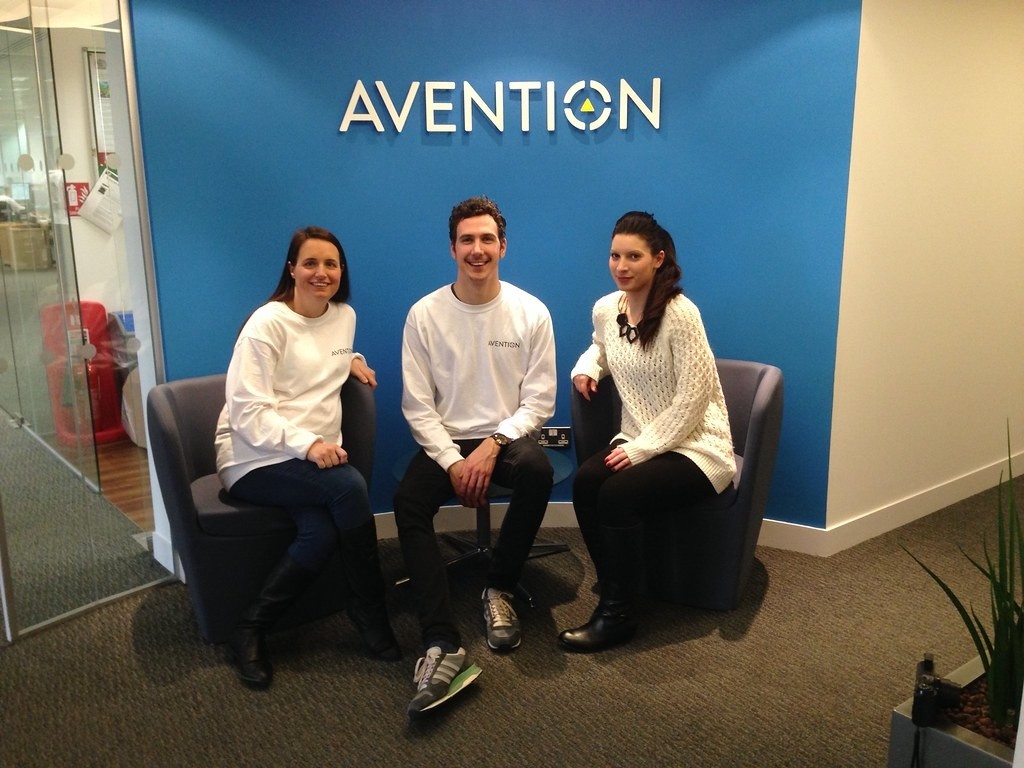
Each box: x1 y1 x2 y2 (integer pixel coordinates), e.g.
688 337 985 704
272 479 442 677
71 354 100 435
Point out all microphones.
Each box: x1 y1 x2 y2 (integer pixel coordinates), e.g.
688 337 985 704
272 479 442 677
616 313 628 327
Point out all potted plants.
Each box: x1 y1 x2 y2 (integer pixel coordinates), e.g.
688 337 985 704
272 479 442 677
889 418 1024 768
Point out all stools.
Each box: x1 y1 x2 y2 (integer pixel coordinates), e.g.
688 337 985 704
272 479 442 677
426 446 571 609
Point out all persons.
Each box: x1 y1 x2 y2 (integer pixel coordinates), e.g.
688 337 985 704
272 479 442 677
395 197 557 716
215 228 398 688
560 210 737 654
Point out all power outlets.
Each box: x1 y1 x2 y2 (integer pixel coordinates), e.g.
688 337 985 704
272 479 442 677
536 426 570 449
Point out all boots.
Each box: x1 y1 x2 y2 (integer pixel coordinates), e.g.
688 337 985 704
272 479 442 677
573 507 604 580
338 521 404 662
227 548 320 683
560 521 646 652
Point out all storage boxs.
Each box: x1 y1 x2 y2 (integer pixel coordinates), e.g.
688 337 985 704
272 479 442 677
0 223 51 272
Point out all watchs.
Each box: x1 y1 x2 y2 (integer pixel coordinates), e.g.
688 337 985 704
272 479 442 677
490 433 508 448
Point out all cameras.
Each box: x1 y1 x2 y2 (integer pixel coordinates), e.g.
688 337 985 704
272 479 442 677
911 653 962 728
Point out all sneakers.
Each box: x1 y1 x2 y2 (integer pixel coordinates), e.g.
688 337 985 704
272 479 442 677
408 645 483 715
479 586 521 650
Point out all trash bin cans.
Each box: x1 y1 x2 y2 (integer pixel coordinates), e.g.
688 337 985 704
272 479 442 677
108 311 139 400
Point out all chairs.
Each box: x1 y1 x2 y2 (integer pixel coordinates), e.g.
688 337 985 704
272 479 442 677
568 359 787 613
149 365 384 642
